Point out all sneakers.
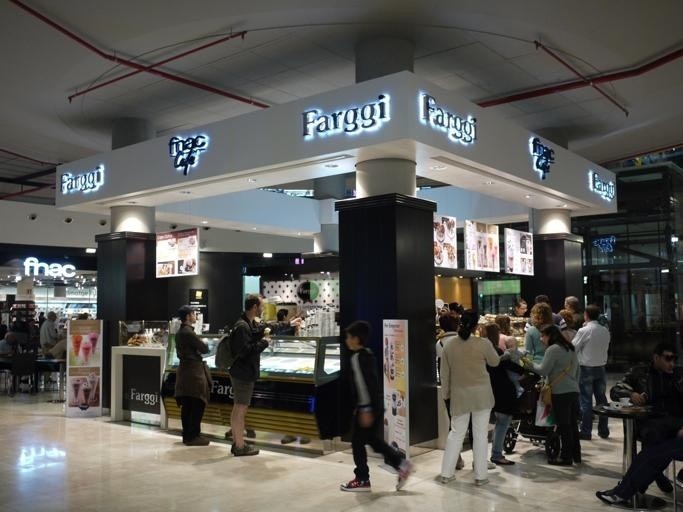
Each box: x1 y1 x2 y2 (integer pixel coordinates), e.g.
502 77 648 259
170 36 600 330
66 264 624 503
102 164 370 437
395 461 418 491
234 444 260 455
596 490 633 508
339 477 372 492
475 478 489 485
491 459 516 466
441 475 456 483
185 435 210 446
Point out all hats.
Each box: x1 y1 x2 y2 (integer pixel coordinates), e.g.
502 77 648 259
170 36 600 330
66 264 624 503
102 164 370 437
178 306 196 320
345 321 369 336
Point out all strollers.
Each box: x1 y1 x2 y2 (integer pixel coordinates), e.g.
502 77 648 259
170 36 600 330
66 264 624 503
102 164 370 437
503 361 560 460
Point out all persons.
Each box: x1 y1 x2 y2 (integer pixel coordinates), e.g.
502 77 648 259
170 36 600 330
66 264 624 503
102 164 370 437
435 312 496 470
559 309 577 343
595 428 683 508
478 323 526 467
522 324 583 466
0 331 18 396
494 315 511 352
339 320 416 492
28 318 39 336
523 295 570 342
500 336 524 399
0 317 7 340
509 299 528 317
272 308 300 337
610 341 683 494
13 312 32 343
39 311 58 384
564 296 584 323
224 295 302 457
174 305 210 446
525 303 553 361
570 304 609 440
39 312 47 329
439 309 500 485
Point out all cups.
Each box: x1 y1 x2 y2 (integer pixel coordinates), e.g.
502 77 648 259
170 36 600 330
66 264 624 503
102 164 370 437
87 332 99 355
83 386 91 405
620 398 630 405
71 379 83 403
71 335 83 357
86 376 98 401
82 342 92 363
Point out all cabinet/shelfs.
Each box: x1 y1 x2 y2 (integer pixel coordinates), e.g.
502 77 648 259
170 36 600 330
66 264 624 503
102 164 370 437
159 333 343 443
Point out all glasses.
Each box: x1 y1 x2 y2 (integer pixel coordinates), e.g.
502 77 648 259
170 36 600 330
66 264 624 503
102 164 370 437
662 354 678 362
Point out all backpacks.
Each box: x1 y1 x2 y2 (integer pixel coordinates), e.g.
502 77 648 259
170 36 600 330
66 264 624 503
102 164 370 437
214 322 250 372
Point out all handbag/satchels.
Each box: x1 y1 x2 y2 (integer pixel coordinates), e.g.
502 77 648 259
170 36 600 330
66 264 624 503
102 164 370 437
541 365 572 404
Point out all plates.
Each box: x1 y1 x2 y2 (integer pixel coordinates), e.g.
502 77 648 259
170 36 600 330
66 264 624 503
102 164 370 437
603 406 620 410
610 401 622 409
622 403 633 406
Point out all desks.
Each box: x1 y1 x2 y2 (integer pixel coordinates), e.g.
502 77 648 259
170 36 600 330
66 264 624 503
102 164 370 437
592 402 667 512
